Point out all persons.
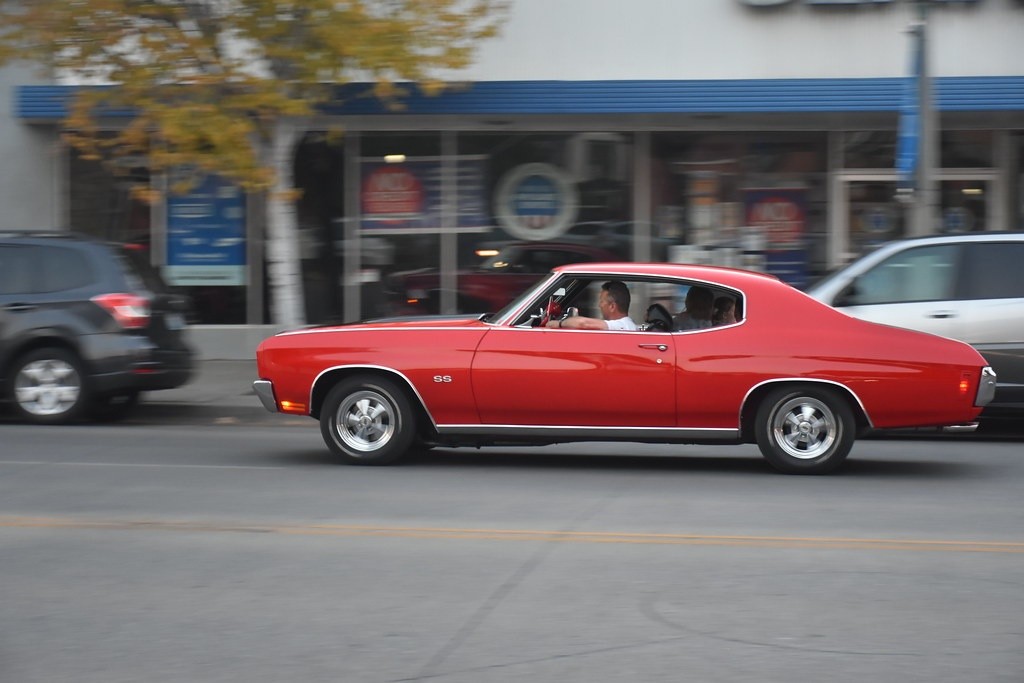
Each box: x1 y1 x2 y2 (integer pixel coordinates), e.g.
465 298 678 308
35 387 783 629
546 280 637 331
670 286 736 333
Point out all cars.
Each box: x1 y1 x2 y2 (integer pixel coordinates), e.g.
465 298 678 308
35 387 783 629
798 234 1024 422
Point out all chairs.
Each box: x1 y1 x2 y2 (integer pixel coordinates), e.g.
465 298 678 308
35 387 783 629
643 303 674 332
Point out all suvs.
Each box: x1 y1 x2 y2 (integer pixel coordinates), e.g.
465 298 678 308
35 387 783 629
0 228 195 427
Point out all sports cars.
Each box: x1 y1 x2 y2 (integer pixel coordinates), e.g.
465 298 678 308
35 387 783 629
362 218 688 317
253 262 998 476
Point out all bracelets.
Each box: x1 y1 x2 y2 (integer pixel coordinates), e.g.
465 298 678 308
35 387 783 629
558 318 564 327
